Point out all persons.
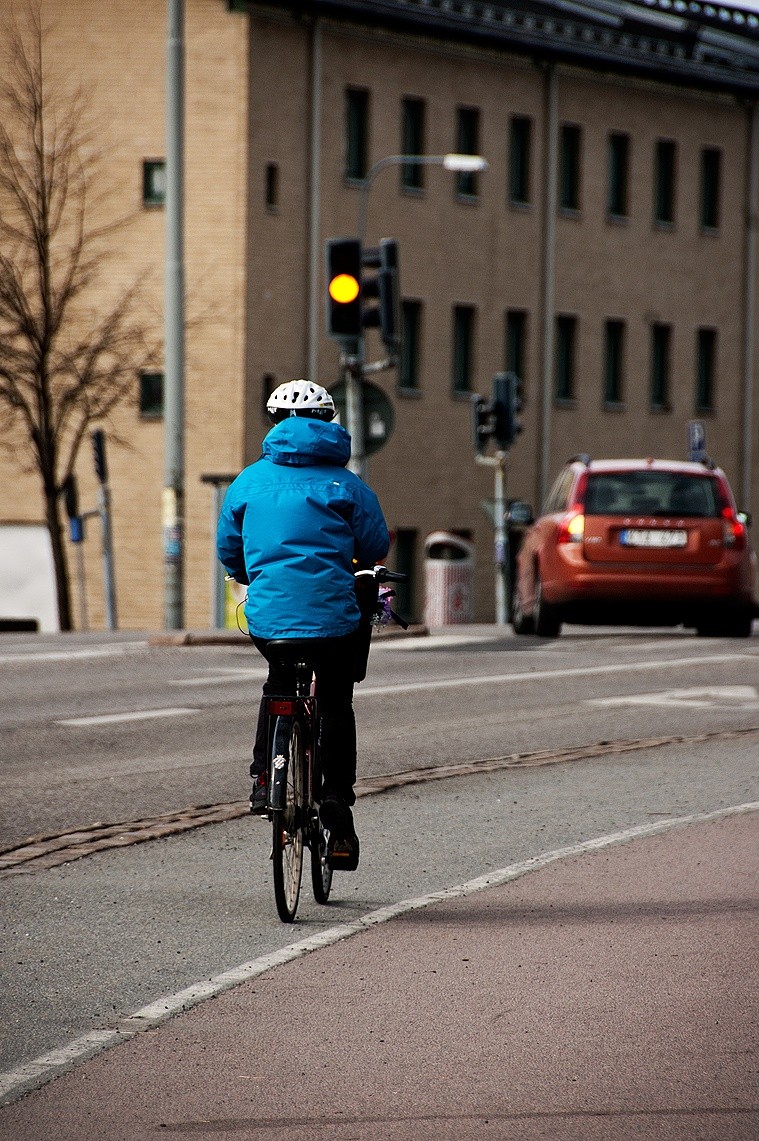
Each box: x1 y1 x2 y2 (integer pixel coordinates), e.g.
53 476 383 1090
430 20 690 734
221 378 391 846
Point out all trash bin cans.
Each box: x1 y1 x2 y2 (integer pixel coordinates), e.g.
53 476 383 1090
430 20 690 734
423 527 475 625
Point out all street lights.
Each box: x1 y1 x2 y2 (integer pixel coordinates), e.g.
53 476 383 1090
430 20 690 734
345 150 488 480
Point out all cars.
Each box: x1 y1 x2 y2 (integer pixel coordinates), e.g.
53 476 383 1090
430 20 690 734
504 453 759 639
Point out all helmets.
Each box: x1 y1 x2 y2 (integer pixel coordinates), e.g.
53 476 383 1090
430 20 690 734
266 379 335 412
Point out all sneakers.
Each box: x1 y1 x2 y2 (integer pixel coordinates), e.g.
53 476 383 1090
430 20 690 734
252 778 269 811
320 795 359 844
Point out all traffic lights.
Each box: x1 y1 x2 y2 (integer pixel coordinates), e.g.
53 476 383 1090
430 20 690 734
471 394 493 457
490 372 524 452
324 238 364 338
363 236 405 353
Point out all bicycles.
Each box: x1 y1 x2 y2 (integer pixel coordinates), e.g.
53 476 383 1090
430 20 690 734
223 563 412 924
